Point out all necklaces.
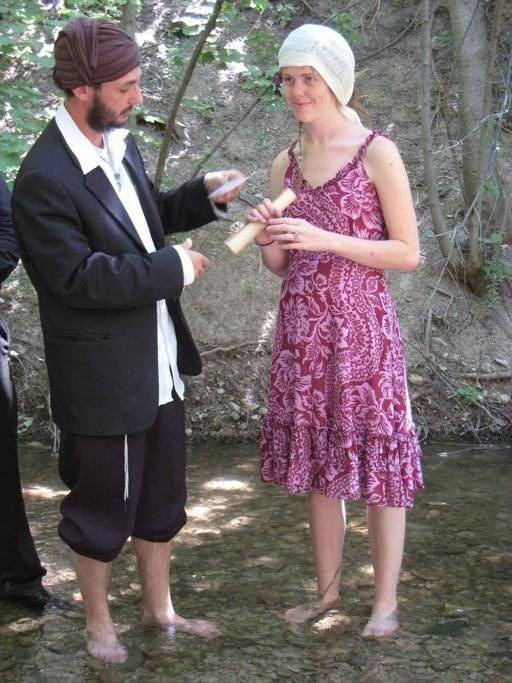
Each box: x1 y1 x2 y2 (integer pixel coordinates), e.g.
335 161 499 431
95 133 122 192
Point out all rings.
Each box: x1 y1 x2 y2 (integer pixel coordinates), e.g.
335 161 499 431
294 234 299 242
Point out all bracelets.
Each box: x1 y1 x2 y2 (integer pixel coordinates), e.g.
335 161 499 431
257 240 276 246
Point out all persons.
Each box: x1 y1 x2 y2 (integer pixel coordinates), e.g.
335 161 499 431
248 23 425 637
10 20 245 665
0 172 70 611
